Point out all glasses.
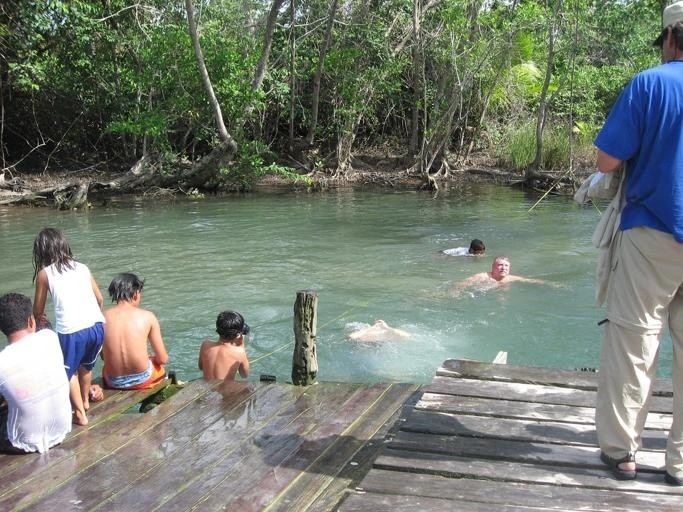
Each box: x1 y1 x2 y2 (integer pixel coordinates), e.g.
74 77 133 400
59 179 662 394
216 324 250 336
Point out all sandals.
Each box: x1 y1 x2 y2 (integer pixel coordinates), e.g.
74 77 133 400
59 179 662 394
600 453 683 486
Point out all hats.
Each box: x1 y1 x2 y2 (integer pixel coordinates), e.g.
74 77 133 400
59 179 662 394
653 1 683 45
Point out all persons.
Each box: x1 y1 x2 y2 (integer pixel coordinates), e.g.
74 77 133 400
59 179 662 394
32 228 106 425
100 273 168 390
35 314 104 403
198 311 250 381
0 293 73 454
461 256 545 285
592 1 683 486
442 239 485 257
